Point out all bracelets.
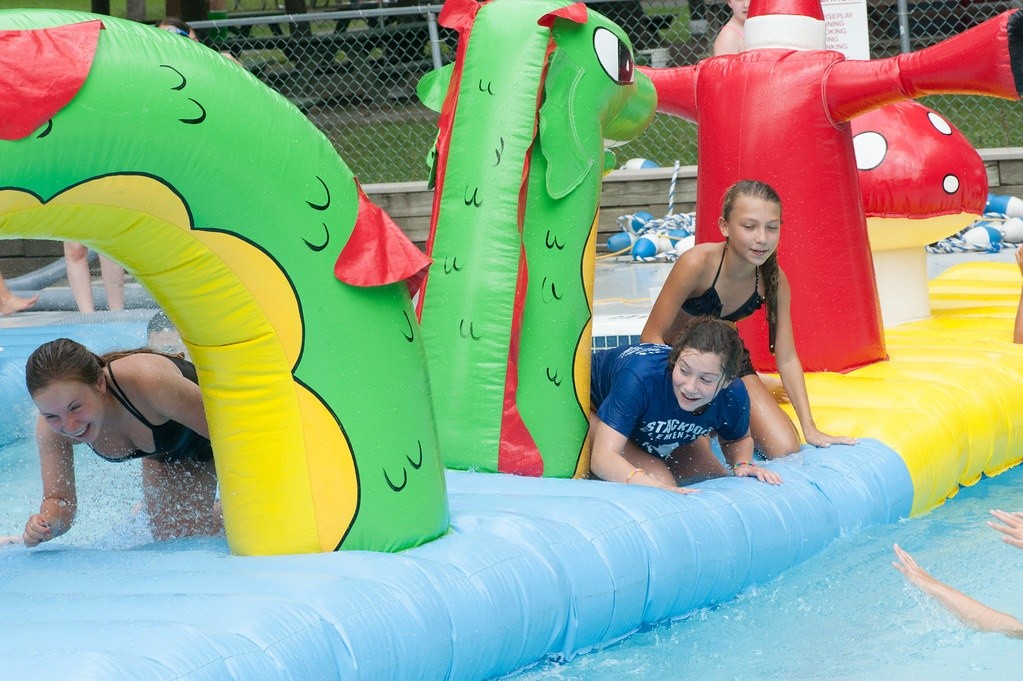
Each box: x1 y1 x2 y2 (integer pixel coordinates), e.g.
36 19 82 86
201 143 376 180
624 468 645 484
733 461 755 472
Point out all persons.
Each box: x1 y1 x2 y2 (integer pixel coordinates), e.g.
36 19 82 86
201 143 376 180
893 509 1023 641
1007 7 1023 345
0 17 244 549
639 178 860 460
714 0 751 56
589 317 785 495
